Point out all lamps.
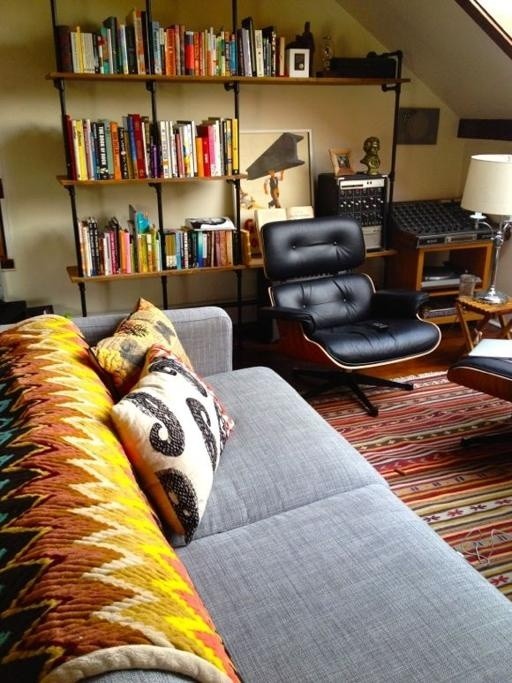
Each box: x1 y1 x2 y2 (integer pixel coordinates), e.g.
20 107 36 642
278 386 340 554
461 154 512 305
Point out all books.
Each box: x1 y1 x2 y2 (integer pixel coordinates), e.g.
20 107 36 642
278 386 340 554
65 113 239 181
74 203 238 278
57 7 285 77
468 339 512 358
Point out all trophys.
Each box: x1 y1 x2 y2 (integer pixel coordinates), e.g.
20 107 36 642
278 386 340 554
319 34 337 78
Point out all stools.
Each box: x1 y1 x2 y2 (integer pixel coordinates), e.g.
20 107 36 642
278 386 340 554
454 295 511 359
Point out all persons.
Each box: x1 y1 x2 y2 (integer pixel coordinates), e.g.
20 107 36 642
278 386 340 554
264 169 283 209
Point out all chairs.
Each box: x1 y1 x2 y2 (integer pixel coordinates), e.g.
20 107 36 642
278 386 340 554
447 339 511 447
260 217 443 415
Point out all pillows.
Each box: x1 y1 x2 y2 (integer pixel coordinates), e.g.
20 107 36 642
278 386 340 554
112 347 236 547
87 297 191 401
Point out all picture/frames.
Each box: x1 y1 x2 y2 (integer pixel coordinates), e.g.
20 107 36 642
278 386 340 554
232 127 314 231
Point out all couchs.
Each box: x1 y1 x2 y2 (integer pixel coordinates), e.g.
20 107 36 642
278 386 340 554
1 304 512 683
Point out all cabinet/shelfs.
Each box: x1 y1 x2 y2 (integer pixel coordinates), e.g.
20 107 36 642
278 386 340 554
387 234 498 325
43 1 413 335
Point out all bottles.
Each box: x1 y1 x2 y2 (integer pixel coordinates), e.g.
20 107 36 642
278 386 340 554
300 21 334 78
